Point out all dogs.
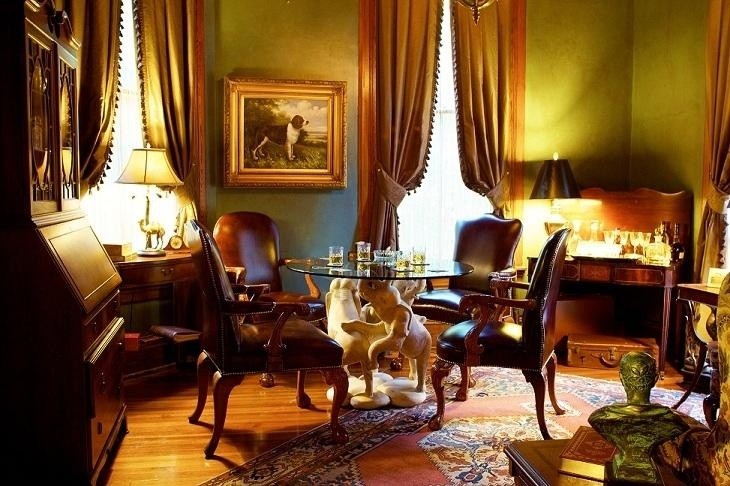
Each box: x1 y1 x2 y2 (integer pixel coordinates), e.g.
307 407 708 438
248 114 310 164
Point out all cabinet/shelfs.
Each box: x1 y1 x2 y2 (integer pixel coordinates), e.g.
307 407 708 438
0 1 128 486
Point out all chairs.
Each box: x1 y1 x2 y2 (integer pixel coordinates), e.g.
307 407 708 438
428 225 578 439
211 211 329 386
389 212 523 387
187 219 352 457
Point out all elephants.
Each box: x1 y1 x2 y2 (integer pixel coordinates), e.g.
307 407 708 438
327 278 432 397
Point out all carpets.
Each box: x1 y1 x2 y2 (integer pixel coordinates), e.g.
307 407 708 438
198 364 708 486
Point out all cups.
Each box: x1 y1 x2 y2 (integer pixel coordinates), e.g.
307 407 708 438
396 250 412 268
357 243 371 261
412 246 427 265
328 245 344 266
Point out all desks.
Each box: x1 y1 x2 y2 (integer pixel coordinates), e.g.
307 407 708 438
673 281 720 427
503 438 663 486
112 249 190 387
525 186 693 376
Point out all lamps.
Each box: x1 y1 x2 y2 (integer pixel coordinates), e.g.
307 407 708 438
530 153 581 247
112 141 186 258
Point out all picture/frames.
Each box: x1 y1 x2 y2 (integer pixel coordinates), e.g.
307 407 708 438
220 73 348 191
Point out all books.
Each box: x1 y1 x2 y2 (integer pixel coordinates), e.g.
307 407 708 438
556 426 619 483
150 325 202 343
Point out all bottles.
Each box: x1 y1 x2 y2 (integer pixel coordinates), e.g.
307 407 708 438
613 227 643 260
646 220 685 264
